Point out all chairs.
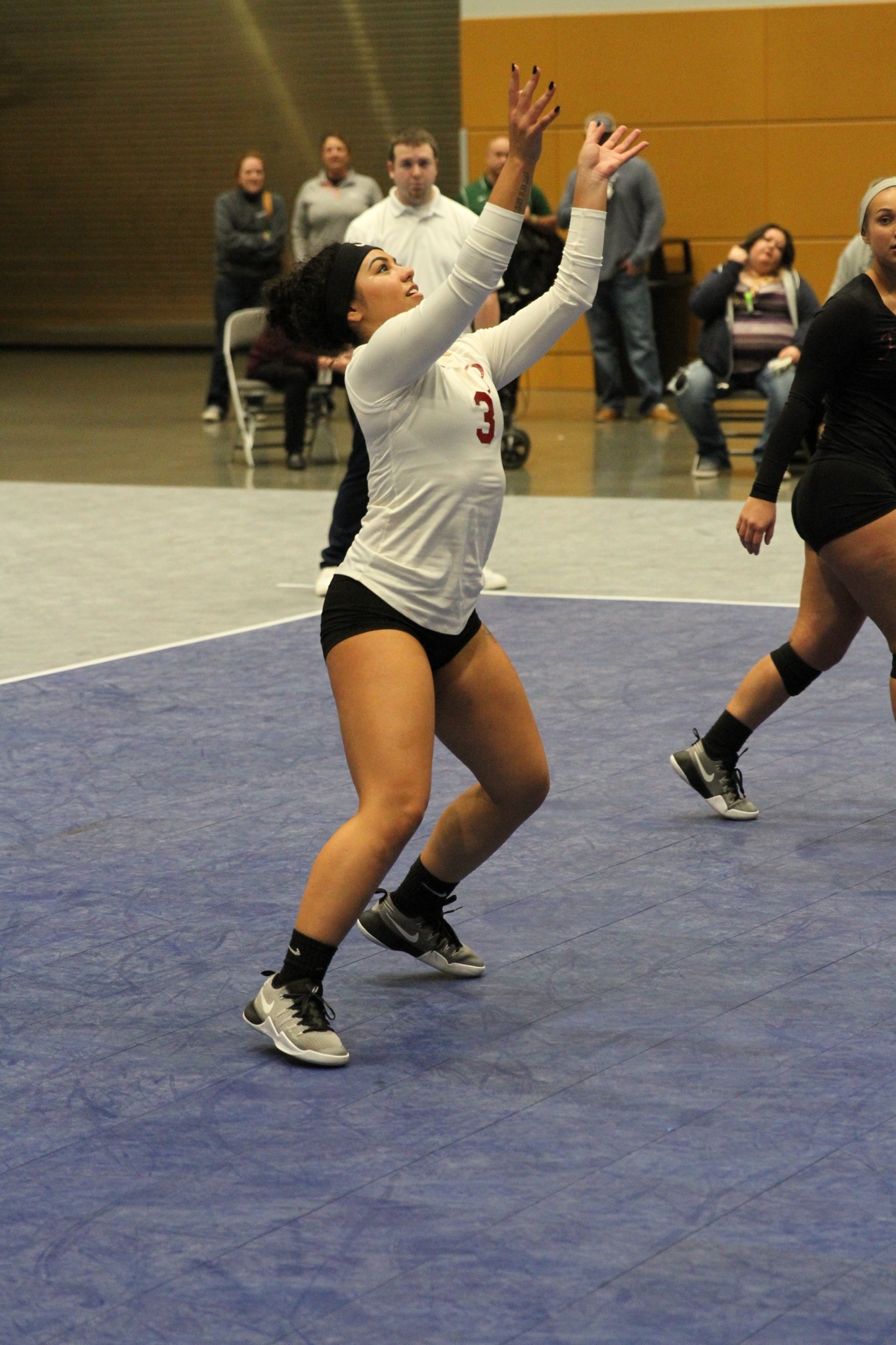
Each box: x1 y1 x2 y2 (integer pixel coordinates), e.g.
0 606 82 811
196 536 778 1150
714 382 769 456
223 307 341 468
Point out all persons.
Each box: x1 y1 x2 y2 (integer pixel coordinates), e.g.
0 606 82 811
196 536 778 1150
669 179 896 821
199 113 880 482
242 61 654 1070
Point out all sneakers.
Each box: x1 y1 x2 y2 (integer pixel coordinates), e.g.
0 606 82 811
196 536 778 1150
670 727 758 820
358 888 486 977
240 971 350 1066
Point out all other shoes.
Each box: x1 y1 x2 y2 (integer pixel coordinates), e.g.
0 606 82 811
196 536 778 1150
696 458 725 477
482 567 507 590
756 464 791 480
595 408 626 422
316 563 339 596
288 453 306 470
203 403 228 421
650 403 678 423
